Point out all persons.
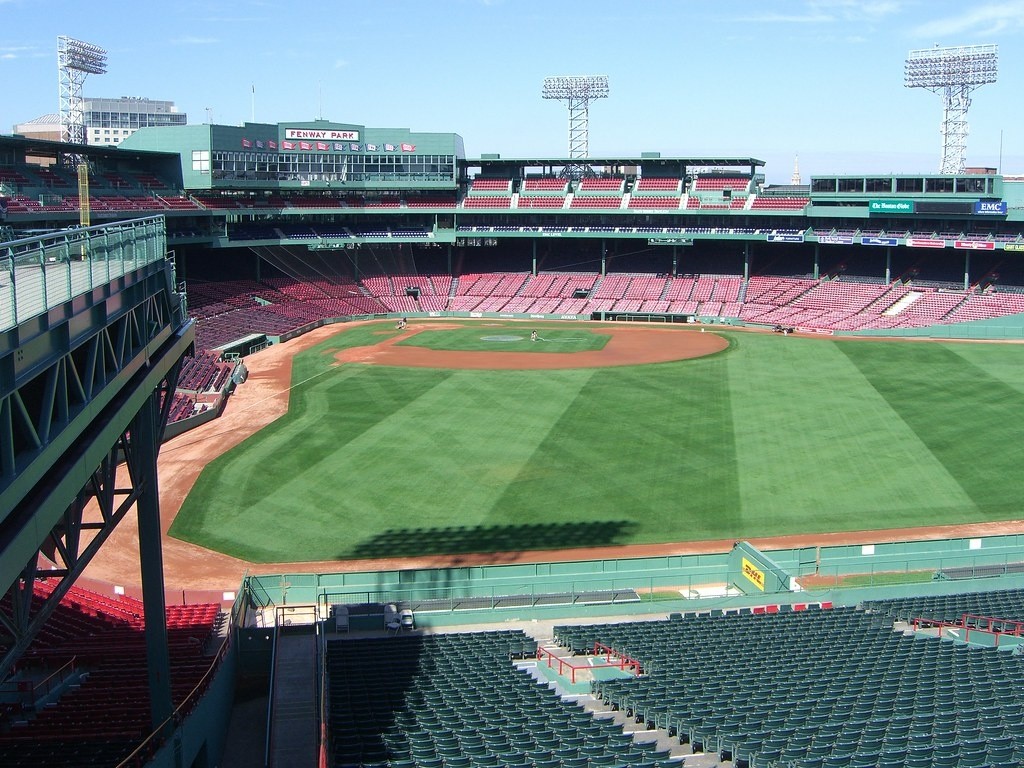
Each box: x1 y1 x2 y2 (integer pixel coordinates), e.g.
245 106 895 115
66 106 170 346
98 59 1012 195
401 317 407 329
531 330 537 339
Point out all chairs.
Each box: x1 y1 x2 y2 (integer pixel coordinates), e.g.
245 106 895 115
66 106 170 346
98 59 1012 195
326 588 1024 768
